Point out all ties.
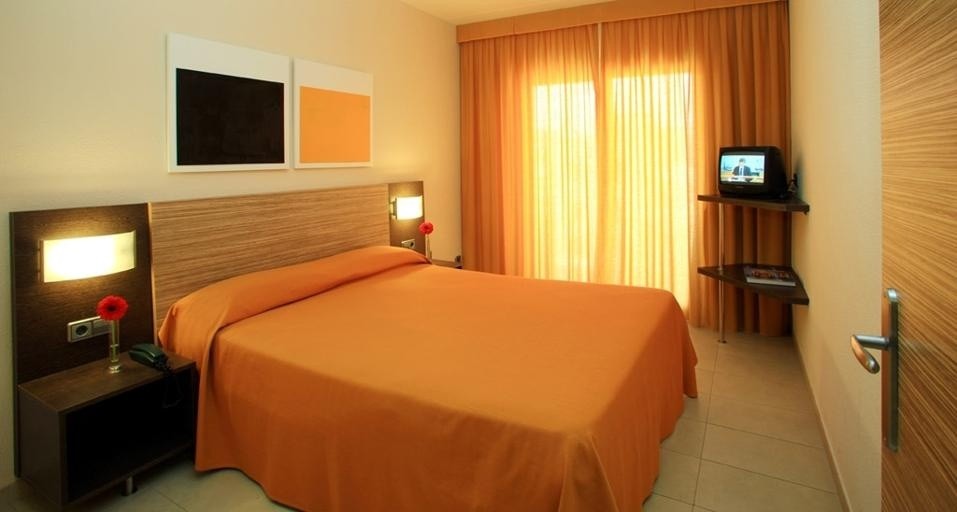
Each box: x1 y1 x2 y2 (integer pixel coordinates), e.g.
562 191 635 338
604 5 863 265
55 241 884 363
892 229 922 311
740 168 743 176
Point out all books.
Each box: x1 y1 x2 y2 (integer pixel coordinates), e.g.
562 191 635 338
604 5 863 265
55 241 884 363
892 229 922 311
744 267 796 286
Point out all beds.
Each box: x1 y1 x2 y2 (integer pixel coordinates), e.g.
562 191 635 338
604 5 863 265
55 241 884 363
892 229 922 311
148 184 697 512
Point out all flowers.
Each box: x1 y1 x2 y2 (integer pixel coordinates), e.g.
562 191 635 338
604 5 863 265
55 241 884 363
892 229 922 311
96 296 129 362
418 222 433 235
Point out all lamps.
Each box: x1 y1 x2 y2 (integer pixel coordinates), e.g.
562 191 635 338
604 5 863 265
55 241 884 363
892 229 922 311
389 194 423 220
34 229 138 285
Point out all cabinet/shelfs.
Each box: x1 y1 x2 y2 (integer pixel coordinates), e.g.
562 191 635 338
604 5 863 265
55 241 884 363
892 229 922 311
696 192 809 344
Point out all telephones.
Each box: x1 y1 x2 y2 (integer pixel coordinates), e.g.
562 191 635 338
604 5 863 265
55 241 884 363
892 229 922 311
128 344 167 366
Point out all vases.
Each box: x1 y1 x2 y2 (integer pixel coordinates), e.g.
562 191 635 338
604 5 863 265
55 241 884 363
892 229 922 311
106 320 124 374
424 235 430 261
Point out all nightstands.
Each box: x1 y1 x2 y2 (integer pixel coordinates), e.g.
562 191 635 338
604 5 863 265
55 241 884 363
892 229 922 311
17 347 197 511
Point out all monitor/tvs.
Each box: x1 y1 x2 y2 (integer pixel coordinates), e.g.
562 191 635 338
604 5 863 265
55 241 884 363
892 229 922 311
717 146 789 200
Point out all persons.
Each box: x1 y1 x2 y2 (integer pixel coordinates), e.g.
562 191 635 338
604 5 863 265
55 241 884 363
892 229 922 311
732 158 752 183
